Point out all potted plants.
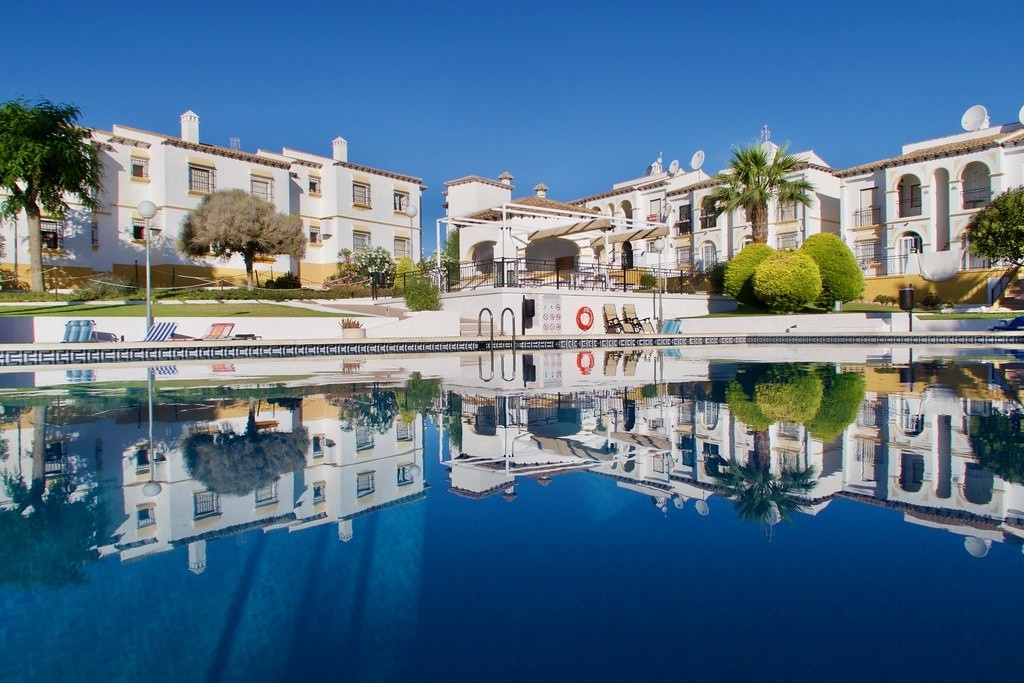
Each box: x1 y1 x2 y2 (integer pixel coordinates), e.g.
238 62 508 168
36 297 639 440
338 318 366 337
339 359 366 375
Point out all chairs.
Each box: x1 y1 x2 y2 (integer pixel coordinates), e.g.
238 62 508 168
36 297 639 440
33 319 98 344
66 369 98 381
602 304 639 333
212 364 235 373
603 350 632 376
166 323 235 341
147 365 179 376
622 304 655 334
623 350 652 376
132 321 177 342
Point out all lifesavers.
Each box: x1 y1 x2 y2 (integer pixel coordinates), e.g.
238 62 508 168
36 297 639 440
576 351 594 375
576 305 595 331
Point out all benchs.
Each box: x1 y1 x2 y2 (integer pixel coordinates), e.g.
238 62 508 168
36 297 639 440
612 282 635 293
580 280 606 291
519 278 545 287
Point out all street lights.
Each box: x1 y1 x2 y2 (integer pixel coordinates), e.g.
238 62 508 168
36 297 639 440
141 367 163 496
5 205 22 287
59 440 72 496
408 410 421 477
655 352 667 435
405 204 417 281
654 239 665 331
138 201 156 332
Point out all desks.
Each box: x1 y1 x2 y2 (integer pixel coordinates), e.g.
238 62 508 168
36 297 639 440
598 274 624 291
569 271 594 289
507 270 533 287
